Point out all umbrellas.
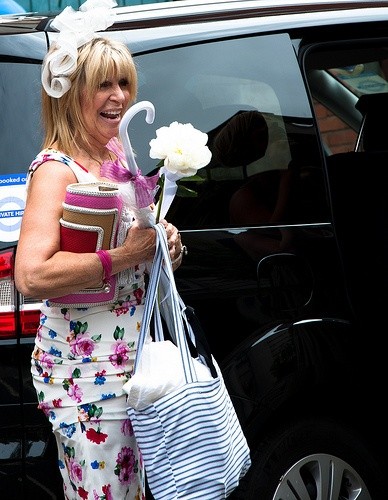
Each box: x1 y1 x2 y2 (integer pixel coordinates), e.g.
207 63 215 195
118 100 192 357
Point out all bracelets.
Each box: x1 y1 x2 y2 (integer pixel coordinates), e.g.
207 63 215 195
95 250 113 280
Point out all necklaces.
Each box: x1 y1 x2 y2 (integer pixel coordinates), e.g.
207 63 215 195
80 143 113 166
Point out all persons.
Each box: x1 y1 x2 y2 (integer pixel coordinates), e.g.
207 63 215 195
13 0 192 500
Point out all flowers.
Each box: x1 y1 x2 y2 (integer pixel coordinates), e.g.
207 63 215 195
149 121 213 224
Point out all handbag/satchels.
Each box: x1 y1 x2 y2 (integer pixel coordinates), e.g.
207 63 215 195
48 182 124 308
126 352 253 500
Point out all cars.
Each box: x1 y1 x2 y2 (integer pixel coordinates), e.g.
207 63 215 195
1 0 388 500
